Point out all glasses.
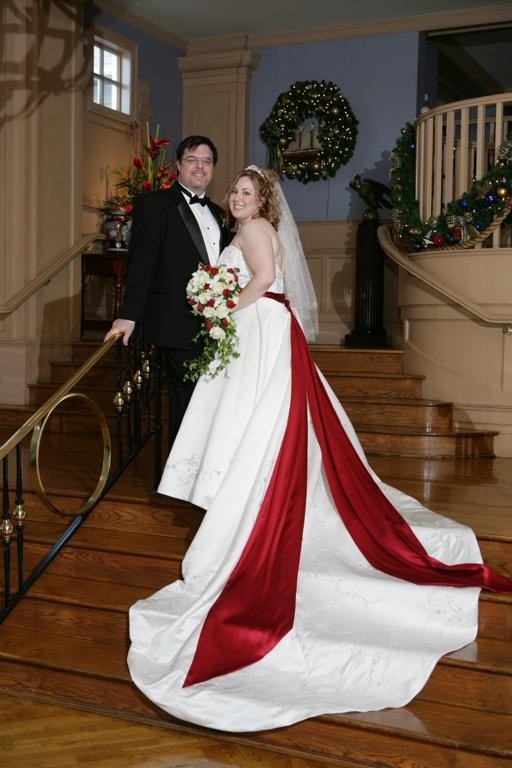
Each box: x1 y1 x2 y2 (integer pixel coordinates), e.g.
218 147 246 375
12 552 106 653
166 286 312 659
182 156 213 165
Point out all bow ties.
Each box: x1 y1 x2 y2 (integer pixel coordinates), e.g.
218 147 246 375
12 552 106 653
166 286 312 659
189 194 210 207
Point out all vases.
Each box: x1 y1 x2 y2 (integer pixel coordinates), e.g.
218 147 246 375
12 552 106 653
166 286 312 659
99 215 127 250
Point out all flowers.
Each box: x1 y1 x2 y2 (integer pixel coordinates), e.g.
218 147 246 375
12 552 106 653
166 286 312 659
180 262 248 382
98 119 178 224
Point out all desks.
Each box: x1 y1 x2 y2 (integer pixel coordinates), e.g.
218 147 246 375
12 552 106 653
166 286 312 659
79 252 133 341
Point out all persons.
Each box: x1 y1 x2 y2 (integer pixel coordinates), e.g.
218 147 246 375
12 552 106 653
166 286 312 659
126 162 510 758
99 134 237 452
